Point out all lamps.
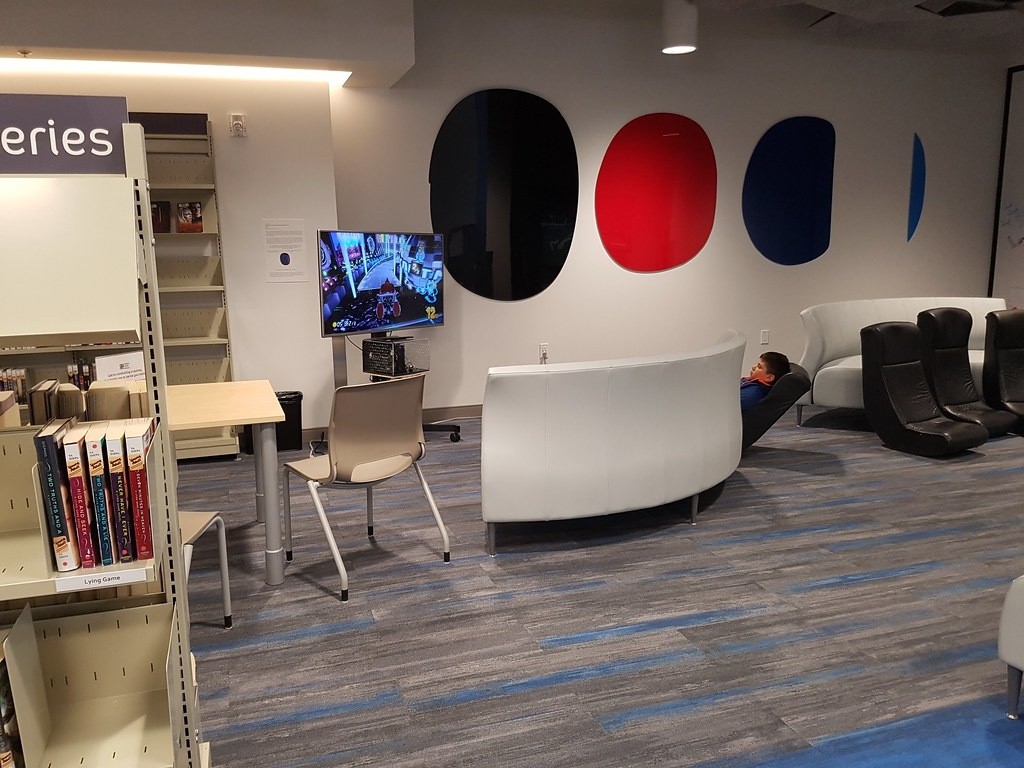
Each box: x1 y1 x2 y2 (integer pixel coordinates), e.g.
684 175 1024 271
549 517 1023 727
662 4 698 55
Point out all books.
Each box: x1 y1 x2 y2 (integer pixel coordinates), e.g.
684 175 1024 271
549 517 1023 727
177 201 203 233
27 378 151 425
0 367 28 416
33 415 155 572
0 628 25 768
65 341 140 349
5 347 36 350
67 350 146 391
0 570 161 612
137 201 171 233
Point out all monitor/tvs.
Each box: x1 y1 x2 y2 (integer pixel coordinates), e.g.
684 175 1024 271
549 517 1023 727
317 229 445 342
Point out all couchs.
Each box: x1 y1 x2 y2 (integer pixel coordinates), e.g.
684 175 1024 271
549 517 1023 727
480 341 747 553
793 297 1014 428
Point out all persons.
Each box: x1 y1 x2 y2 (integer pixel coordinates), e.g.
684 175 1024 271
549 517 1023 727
178 203 202 224
741 352 791 411
60 484 72 526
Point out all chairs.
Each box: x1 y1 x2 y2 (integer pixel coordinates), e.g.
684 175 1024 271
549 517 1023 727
281 373 451 600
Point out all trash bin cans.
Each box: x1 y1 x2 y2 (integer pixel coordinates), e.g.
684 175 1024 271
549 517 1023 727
239 390 303 455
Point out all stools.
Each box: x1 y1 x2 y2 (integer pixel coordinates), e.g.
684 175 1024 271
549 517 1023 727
179 508 233 630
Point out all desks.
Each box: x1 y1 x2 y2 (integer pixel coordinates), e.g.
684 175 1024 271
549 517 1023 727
166 378 288 584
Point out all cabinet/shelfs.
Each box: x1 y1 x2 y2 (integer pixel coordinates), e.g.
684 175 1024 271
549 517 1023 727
130 112 241 461
1 79 215 768
